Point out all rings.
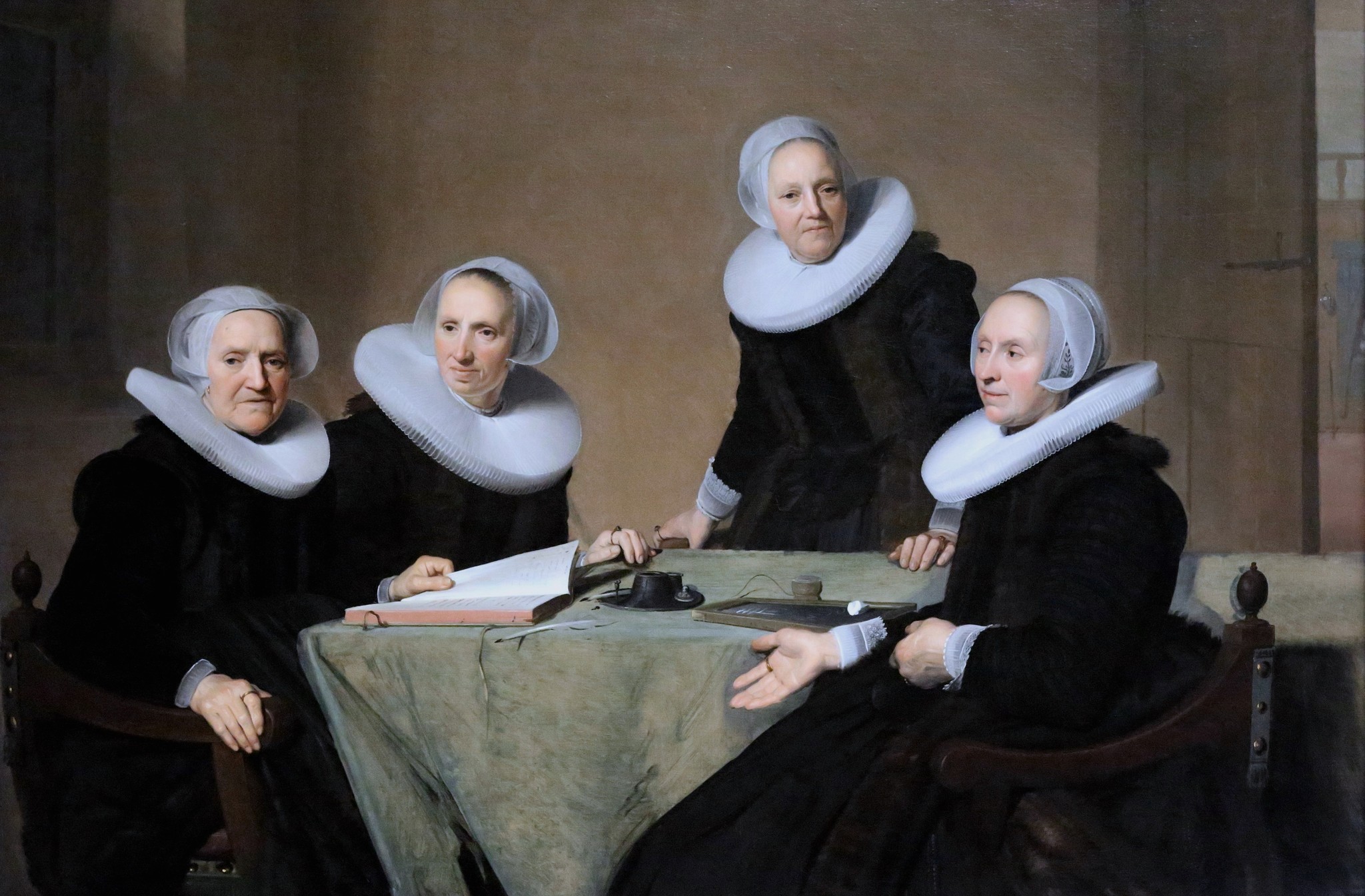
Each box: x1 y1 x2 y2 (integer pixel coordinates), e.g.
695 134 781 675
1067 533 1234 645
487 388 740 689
241 691 259 700
610 526 622 545
765 657 773 672
935 536 946 549
654 525 665 541
904 679 912 686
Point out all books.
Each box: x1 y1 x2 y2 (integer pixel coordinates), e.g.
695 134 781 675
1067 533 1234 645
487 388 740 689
341 540 587 626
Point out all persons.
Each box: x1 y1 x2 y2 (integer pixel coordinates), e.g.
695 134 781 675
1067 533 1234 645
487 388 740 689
324 256 657 564
35 285 454 896
607 276 1189 896
655 114 985 573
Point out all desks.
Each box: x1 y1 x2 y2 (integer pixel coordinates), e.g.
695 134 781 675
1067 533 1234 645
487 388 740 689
301 551 1364 896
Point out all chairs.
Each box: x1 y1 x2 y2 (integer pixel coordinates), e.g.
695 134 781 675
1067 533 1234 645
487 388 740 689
1 550 293 896
880 561 1277 895
